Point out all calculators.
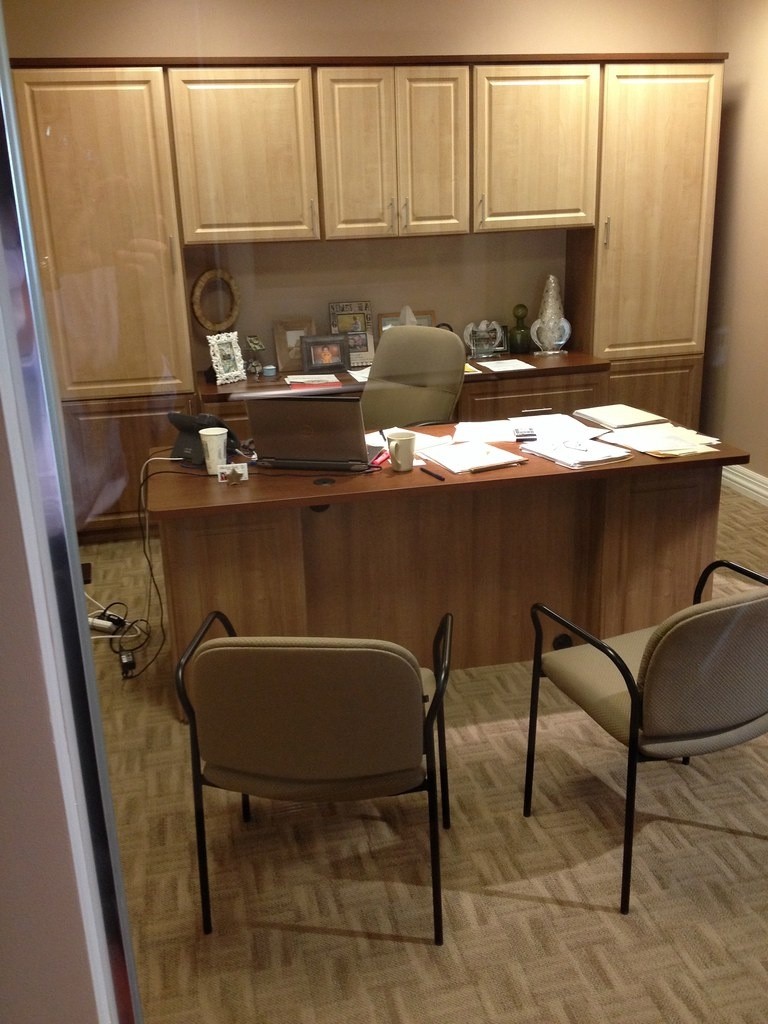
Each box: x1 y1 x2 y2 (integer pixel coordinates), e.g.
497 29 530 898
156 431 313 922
513 426 538 441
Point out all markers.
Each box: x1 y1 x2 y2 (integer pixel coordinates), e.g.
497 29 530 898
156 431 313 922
373 452 390 466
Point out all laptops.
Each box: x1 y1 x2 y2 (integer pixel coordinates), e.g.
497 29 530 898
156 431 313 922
243 395 385 472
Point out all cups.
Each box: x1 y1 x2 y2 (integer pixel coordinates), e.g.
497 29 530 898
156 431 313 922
199 427 228 475
387 431 415 472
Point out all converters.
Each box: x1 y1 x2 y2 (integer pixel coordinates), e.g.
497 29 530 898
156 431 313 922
120 651 135 671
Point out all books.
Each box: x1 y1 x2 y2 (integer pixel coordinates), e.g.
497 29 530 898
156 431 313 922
422 404 669 476
283 375 342 390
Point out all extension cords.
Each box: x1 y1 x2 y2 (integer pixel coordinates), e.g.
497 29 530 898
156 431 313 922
87 618 116 635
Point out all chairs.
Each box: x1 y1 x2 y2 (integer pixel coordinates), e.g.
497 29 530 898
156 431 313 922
174 610 454 948
521 559 768 919
358 325 467 432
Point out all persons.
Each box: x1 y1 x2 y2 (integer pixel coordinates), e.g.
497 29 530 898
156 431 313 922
351 315 361 331
220 473 227 480
321 345 332 363
349 334 362 349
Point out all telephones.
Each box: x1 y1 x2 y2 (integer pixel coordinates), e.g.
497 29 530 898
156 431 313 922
167 411 241 465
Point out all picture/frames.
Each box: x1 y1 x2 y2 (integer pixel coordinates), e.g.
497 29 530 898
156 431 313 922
377 310 436 343
329 300 375 367
272 318 317 372
205 331 247 385
299 331 352 375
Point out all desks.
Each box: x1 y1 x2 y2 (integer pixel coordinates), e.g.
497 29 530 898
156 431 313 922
146 405 750 672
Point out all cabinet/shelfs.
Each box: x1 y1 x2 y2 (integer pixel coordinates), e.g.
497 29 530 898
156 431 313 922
314 66 471 240
565 62 725 357
166 65 323 246
10 66 196 402
470 64 602 233
61 355 704 545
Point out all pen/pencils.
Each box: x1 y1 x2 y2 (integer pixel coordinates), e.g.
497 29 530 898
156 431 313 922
419 467 445 482
